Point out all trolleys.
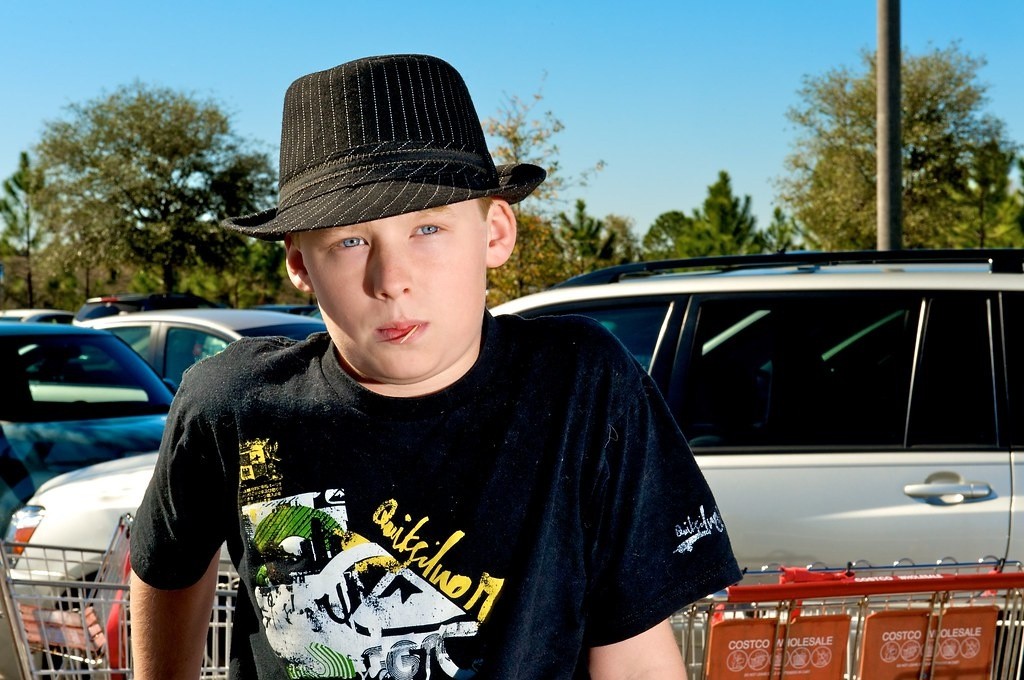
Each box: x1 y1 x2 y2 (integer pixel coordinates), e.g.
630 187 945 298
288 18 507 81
0 512 238 680
670 555 1024 680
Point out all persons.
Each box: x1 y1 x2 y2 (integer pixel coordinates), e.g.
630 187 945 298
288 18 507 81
129 54 745 680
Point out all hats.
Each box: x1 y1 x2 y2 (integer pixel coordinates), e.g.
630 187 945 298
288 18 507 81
220 53 547 241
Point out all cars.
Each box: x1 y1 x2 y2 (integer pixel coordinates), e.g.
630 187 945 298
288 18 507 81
0 292 327 680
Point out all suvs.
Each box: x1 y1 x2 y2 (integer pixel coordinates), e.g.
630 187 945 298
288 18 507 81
488 245 1024 583
